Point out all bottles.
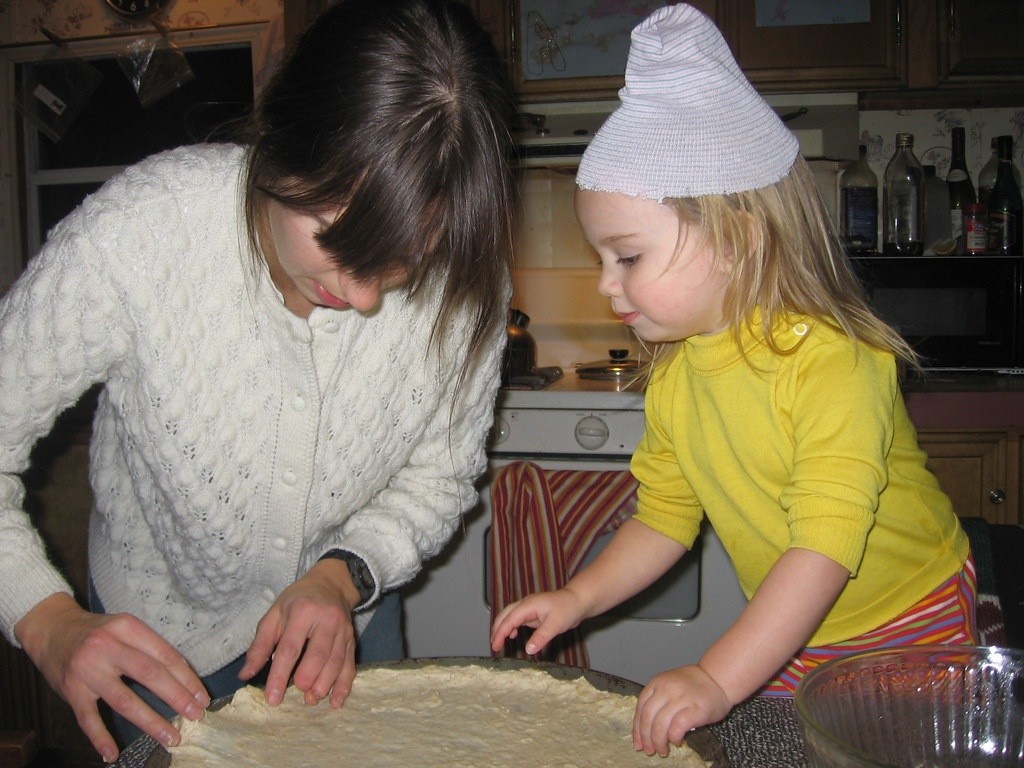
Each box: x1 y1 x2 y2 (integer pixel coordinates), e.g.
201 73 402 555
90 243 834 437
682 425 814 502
978 137 1022 209
989 135 1023 255
967 204 988 256
946 128 976 257
922 165 952 256
839 144 878 249
882 133 924 256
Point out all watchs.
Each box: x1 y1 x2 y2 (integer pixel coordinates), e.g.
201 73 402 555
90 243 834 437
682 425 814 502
316 549 376 609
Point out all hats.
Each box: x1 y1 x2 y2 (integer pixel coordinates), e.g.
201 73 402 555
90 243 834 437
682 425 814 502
575 2 801 200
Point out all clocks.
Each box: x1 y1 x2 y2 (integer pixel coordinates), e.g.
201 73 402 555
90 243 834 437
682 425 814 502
103 0 176 24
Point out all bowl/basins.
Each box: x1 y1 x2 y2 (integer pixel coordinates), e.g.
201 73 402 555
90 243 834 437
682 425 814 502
795 643 1024 767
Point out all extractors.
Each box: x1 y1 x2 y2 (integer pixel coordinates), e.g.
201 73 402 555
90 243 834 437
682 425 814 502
511 111 613 169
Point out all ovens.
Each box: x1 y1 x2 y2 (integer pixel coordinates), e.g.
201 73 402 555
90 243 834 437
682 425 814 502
404 459 747 686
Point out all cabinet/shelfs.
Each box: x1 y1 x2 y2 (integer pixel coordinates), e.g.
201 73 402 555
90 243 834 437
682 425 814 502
469 0 1024 110
903 392 1024 528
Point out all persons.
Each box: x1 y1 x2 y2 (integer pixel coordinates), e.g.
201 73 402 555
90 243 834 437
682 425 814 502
1 0 526 762
487 0 978 758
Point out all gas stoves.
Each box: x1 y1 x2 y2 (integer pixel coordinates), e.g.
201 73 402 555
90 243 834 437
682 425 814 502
484 366 646 454
